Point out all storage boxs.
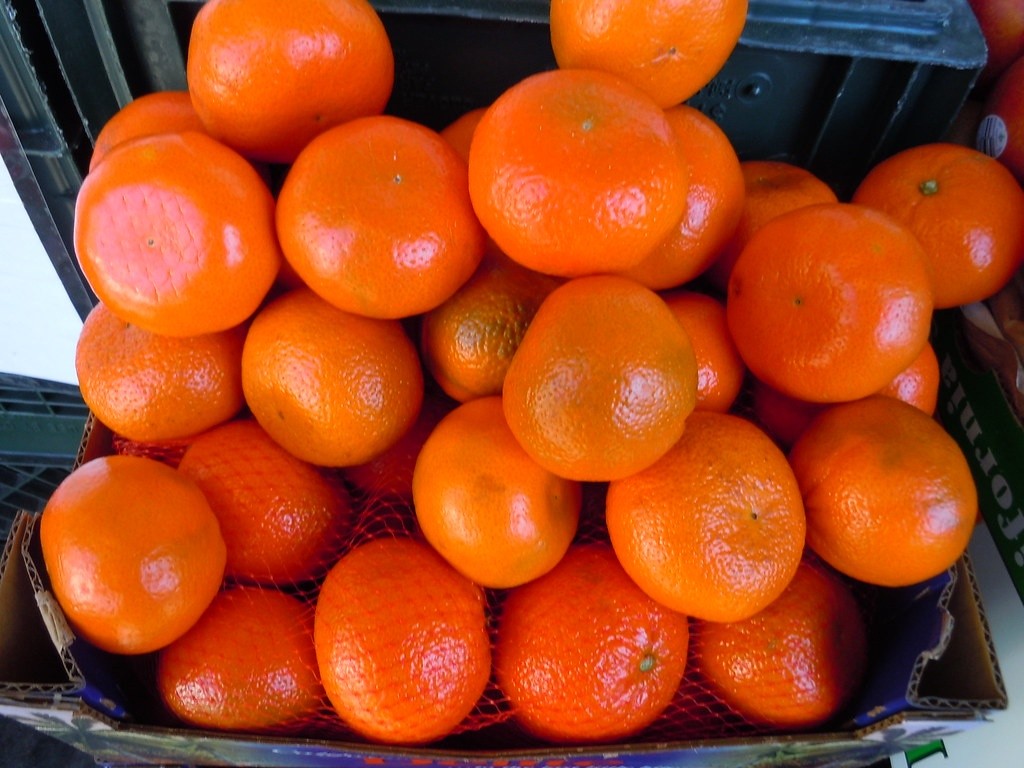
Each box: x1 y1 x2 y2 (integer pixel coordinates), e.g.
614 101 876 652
0 399 1011 768
935 296 1024 604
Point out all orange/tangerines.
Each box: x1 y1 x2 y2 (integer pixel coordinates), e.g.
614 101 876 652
6 1 1024 764
